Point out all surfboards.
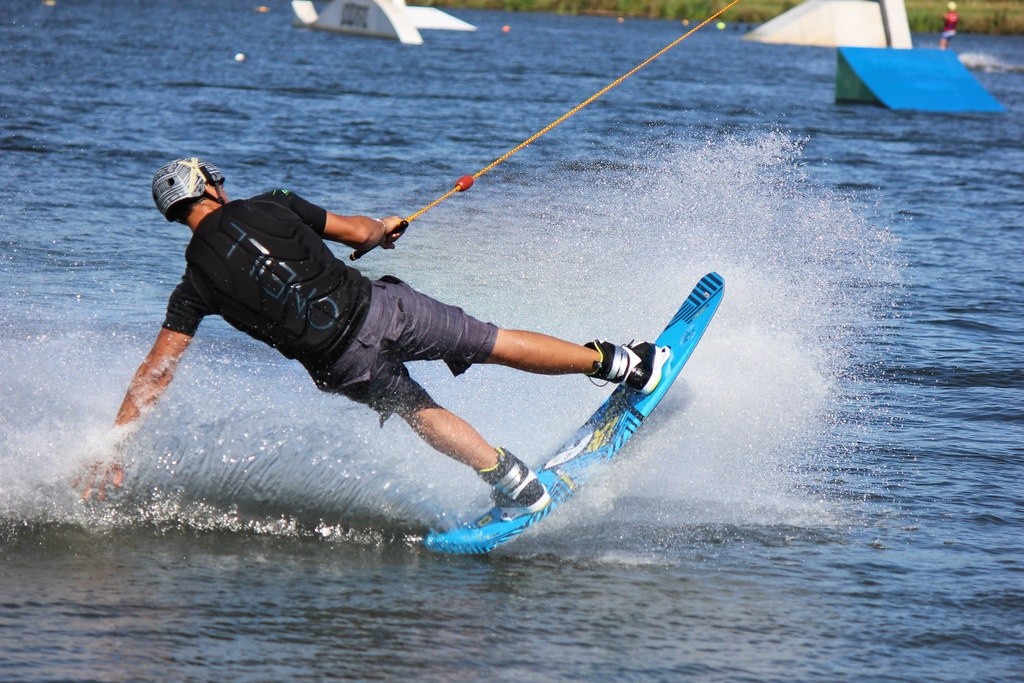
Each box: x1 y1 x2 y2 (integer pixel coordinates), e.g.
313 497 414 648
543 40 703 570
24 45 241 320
423 271 726 556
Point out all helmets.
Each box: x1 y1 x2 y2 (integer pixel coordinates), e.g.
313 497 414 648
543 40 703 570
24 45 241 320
153 157 226 224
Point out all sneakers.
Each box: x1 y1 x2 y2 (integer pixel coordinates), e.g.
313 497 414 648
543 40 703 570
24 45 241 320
478 448 542 509
583 340 655 389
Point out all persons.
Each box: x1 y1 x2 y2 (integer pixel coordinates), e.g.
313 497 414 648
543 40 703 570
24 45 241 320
71 158 671 524
939 1 961 51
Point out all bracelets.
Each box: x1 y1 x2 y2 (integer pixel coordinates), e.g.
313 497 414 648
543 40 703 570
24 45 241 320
374 218 387 236
